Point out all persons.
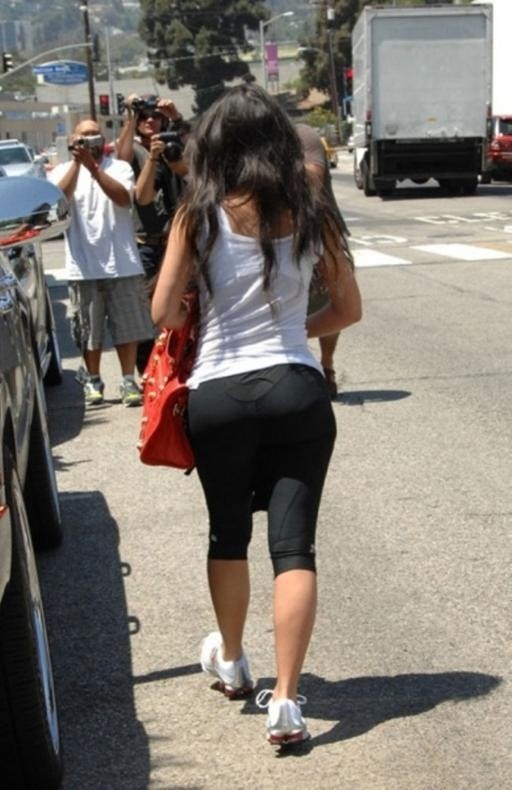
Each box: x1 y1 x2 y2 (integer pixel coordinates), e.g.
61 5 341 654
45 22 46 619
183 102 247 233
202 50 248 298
291 119 354 398
116 98 195 386
148 86 362 747
48 119 152 407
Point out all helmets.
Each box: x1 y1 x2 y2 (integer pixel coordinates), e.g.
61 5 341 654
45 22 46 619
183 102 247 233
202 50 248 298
135 93 169 133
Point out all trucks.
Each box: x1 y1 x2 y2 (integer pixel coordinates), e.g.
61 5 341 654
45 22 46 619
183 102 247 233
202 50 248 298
347 6 494 198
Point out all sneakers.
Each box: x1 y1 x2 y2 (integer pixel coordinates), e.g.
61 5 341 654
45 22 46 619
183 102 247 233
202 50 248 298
199 632 253 697
255 689 310 746
119 379 143 407
83 375 105 406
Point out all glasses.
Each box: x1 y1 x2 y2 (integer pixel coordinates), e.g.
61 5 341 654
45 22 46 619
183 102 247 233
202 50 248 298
139 112 161 120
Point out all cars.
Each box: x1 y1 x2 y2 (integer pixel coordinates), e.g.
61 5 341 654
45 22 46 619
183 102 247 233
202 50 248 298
0 138 48 182
5 239 65 386
314 136 339 166
0 167 69 787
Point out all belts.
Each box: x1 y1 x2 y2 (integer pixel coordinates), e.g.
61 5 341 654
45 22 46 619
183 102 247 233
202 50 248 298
137 237 166 246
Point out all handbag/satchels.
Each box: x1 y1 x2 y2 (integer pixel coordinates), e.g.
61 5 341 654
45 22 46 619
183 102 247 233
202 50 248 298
136 295 194 468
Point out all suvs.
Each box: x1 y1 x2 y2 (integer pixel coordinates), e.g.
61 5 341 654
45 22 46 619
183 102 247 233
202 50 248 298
483 115 512 183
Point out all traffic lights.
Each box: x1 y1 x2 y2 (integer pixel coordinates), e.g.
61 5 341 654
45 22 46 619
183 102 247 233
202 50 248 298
100 94 109 116
118 93 127 115
3 51 14 76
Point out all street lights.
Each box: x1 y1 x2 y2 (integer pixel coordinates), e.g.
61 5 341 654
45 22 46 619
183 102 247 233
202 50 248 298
258 12 294 102
324 7 344 147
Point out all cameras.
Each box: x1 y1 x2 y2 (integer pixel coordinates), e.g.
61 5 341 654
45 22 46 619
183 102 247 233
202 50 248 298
67 134 104 153
158 131 184 162
130 94 158 113
